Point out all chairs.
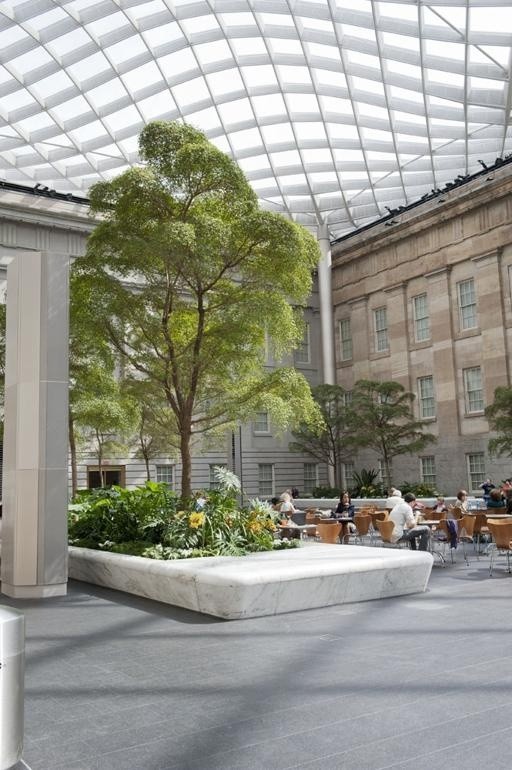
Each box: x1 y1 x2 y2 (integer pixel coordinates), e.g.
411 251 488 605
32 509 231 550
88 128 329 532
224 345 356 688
273 503 512 578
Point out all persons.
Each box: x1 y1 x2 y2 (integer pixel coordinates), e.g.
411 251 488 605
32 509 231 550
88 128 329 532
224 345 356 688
331 490 356 543
386 479 512 551
265 487 298 528
